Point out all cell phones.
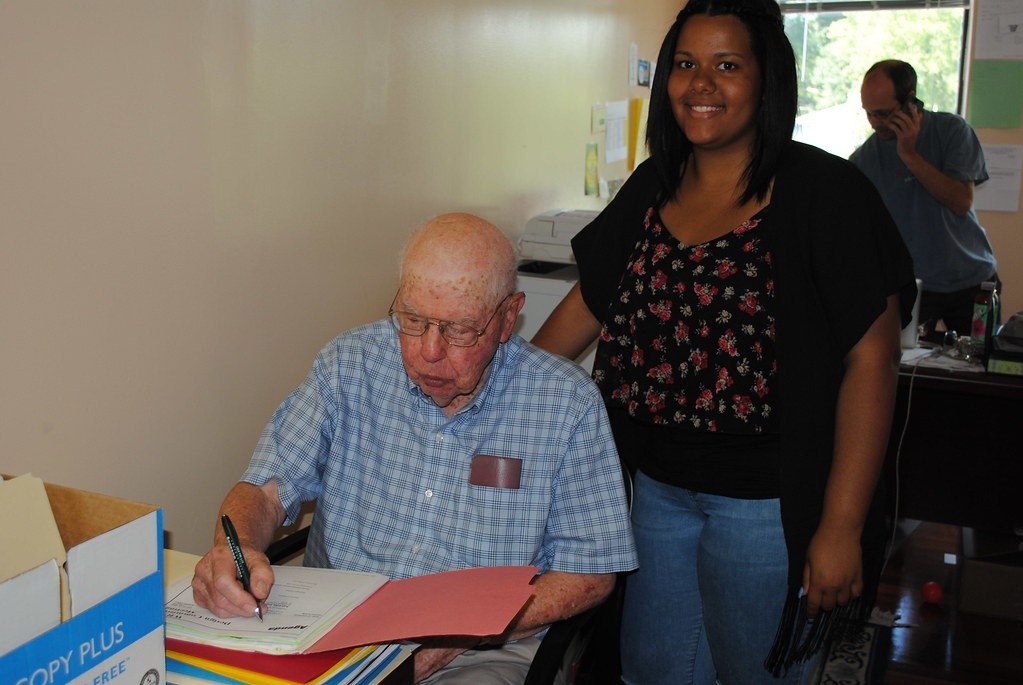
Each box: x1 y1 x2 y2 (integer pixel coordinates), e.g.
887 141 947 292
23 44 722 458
889 98 924 133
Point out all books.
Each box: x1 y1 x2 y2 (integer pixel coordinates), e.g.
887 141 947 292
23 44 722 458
162 549 540 685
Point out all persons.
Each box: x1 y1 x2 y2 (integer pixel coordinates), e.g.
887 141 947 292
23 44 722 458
530 0 918 685
191 213 640 685
849 60 1002 343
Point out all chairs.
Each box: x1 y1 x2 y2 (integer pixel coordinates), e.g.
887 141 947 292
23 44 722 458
271 447 634 685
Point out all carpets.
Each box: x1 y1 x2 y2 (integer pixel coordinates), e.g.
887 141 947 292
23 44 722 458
791 600 919 685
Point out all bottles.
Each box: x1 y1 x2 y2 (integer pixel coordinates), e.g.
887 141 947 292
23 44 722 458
970 282 999 358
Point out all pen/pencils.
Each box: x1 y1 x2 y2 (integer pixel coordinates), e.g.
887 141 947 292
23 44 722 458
221 513 265 622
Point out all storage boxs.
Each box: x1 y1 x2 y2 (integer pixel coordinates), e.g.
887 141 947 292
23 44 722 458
0 476 169 685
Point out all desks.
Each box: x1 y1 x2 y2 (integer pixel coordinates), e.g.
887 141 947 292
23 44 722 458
884 329 1023 568
162 548 414 685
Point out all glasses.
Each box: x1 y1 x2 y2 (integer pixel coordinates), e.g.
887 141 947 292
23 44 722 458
867 96 907 120
388 288 512 348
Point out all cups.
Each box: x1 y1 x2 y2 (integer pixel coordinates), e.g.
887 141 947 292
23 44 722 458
900 278 922 348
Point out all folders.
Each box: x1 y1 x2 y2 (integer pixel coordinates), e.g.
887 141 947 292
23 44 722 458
165 635 410 685
164 564 542 656
